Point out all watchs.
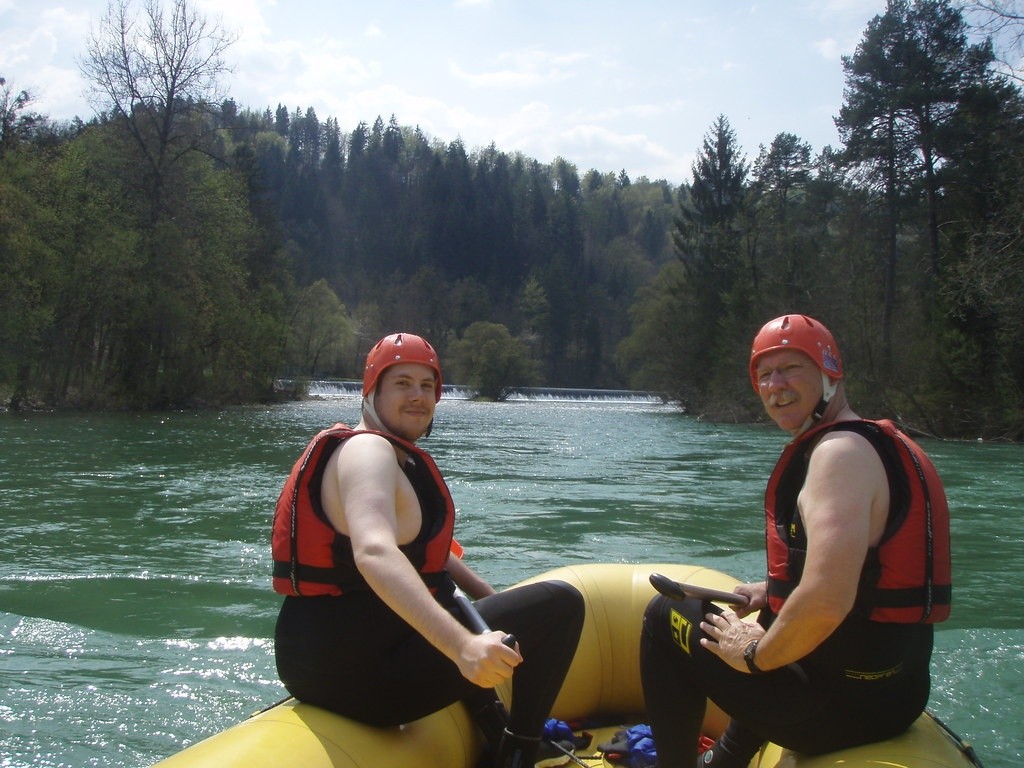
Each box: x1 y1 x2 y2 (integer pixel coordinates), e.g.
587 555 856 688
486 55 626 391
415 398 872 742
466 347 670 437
743 639 762 676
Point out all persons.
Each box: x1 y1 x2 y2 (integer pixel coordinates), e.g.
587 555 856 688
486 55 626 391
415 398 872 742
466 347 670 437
271 332 587 768
635 312 952 768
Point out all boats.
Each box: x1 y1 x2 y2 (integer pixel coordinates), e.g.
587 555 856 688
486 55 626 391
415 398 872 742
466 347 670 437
150 562 984 768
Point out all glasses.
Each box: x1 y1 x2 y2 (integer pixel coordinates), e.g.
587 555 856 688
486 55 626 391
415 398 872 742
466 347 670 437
753 359 813 384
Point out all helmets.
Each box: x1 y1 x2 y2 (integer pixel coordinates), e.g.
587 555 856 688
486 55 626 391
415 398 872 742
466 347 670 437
362 332 442 404
748 314 843 396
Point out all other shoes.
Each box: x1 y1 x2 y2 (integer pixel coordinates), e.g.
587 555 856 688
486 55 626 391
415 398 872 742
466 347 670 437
473 698 594 768
